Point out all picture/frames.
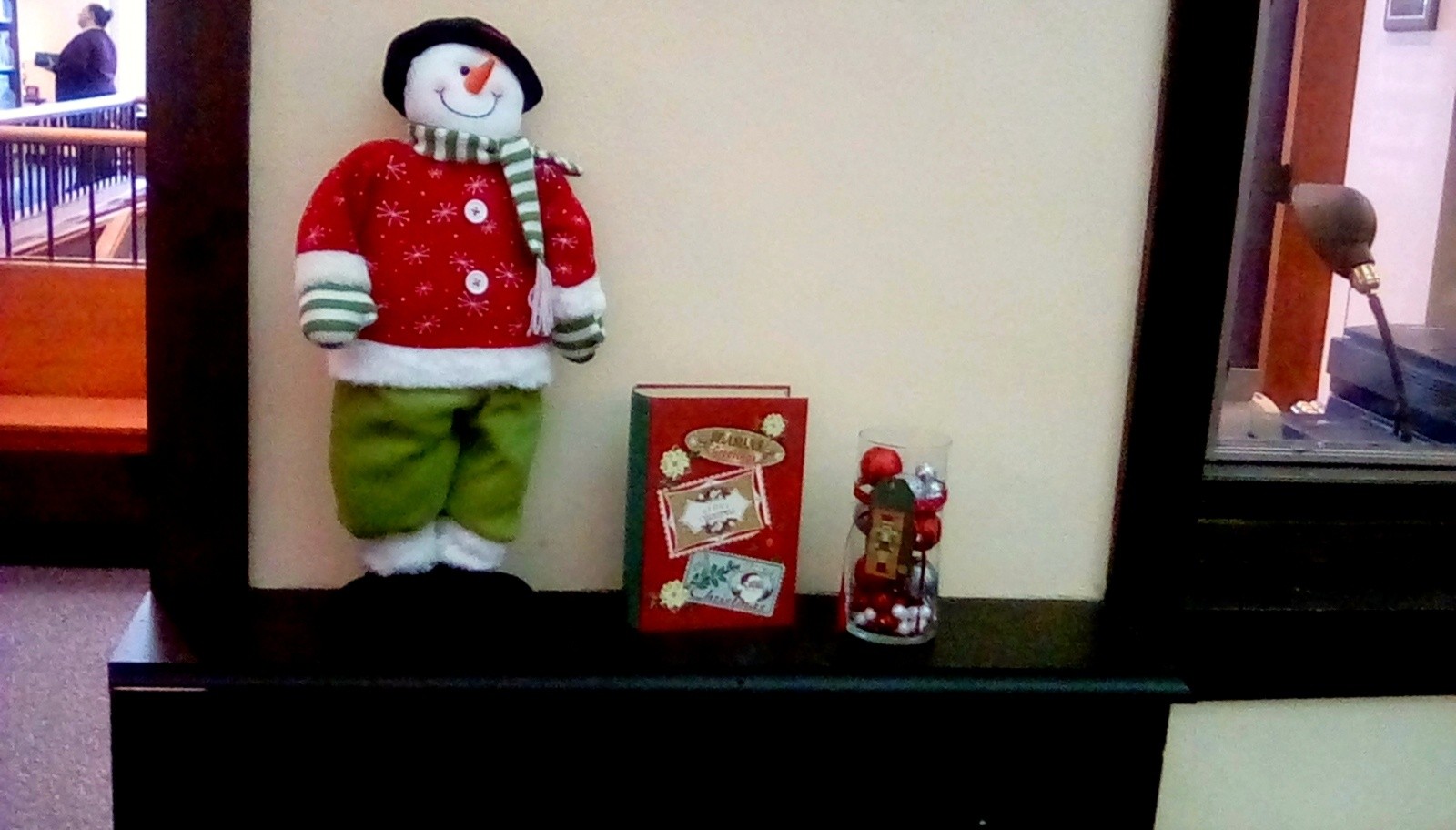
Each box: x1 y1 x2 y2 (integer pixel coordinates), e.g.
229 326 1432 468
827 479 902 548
1384 0 1438 32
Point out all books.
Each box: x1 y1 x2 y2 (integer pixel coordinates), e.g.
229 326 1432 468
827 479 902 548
622 381 809 632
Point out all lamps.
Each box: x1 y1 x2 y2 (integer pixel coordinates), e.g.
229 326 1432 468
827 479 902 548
1290 182 1412 446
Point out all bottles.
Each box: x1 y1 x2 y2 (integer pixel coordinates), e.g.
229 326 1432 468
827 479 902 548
836 428 952 646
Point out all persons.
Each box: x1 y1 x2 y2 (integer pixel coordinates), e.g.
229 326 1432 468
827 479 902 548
296 17 606 628
41 4 117 193
